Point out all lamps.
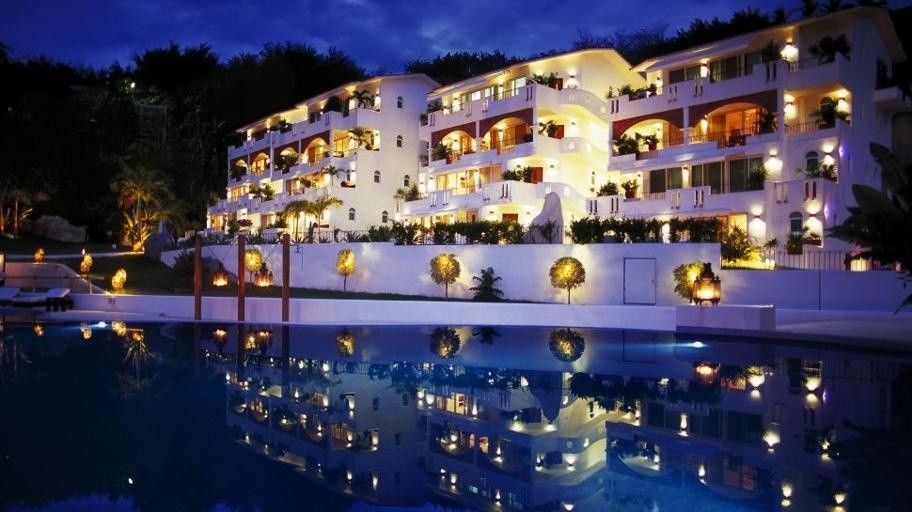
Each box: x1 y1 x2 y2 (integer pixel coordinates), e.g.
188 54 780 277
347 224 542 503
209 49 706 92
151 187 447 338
336 249 356 292
211 247 274 288
430 254 460 298
674 261 721 306
550 256 585 306
33 248 128 296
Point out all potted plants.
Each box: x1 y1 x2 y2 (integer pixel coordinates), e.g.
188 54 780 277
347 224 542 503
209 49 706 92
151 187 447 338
394 73 563 212
206 89 381 234
